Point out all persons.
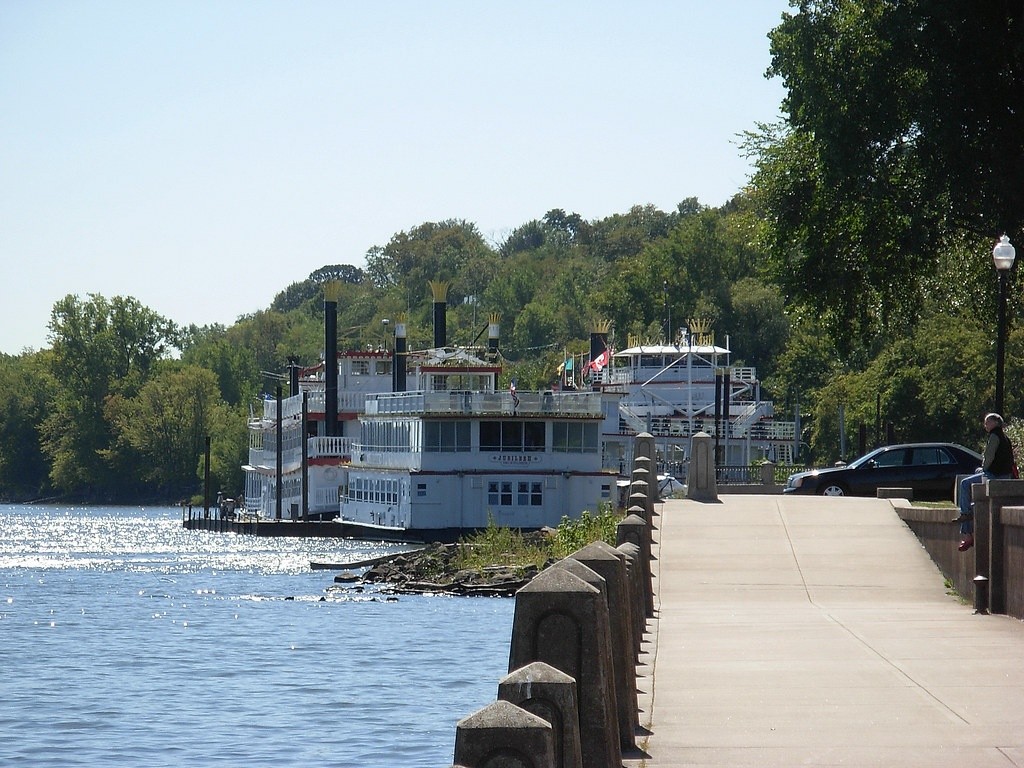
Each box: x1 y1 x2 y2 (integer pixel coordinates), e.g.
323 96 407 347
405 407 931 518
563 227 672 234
950 414 1015 551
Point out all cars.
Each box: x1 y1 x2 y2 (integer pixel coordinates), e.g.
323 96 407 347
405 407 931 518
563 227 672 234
783 443 1018 496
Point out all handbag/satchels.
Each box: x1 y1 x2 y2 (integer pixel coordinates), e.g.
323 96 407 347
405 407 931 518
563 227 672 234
1012 463 1019 479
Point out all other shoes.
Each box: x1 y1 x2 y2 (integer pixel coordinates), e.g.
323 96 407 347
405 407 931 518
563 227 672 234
959 537 974 552
952 516 962 524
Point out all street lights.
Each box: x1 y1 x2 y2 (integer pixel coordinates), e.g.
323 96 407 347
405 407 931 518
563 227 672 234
992 233 1021 422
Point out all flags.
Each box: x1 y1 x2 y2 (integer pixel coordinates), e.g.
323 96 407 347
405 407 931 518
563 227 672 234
510 377 519 408
556 350 609 377
